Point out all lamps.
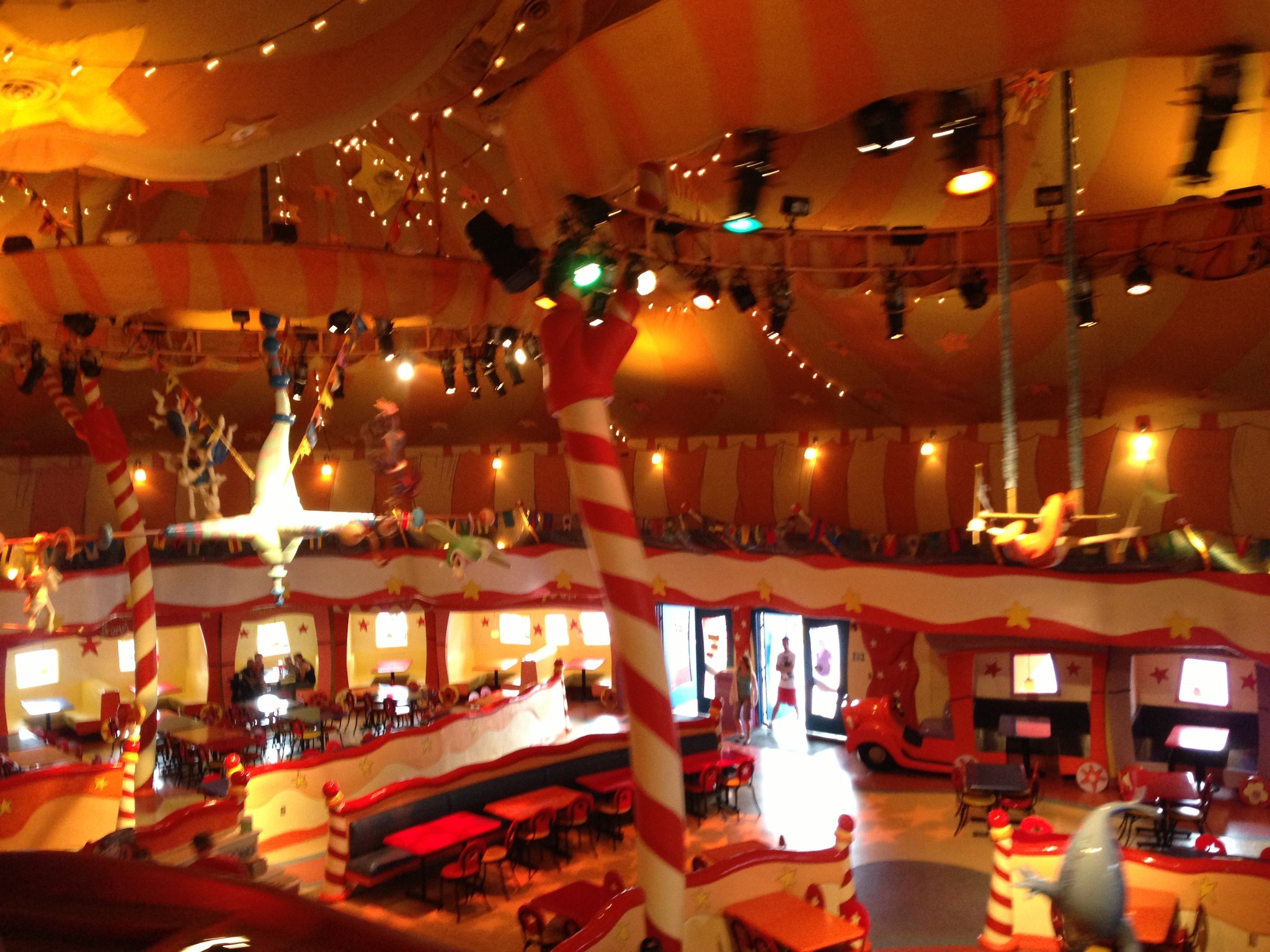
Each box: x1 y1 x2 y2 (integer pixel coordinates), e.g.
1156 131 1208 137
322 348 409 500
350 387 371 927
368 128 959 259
17 342 101 398
849 89 1154 340
438 127 810 401
232 311 395 401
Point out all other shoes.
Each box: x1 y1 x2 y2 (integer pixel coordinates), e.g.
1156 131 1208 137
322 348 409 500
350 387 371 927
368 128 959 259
735 735 745 743
743 740 751 746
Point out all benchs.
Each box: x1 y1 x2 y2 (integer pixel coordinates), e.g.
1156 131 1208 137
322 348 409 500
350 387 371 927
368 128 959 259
91 677 130 731
63 678 111 744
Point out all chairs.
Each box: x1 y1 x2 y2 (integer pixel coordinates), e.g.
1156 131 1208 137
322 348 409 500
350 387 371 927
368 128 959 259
23 693 410 801
952 756 1213 849
439 759 872 952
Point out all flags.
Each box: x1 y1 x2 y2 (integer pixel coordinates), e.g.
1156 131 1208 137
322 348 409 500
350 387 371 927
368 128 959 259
444 506 572 536
153 534 242 556
806 519 961 557
642 515 787 546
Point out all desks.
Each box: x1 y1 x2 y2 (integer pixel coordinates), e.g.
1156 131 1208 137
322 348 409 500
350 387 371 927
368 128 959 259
723 891 867 952
1132 726 1230 850
682 751 756 818
383 810 501 910
530 880 614 929
576 768 634 842
21 658 607 794
970 715 1051 838
484 785 594 869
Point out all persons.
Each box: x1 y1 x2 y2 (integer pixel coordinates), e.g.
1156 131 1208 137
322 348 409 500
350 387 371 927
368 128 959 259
231 653 268 704
730 654 759 746
188 833 251 884
763 636 799 729
283 652 315 701
